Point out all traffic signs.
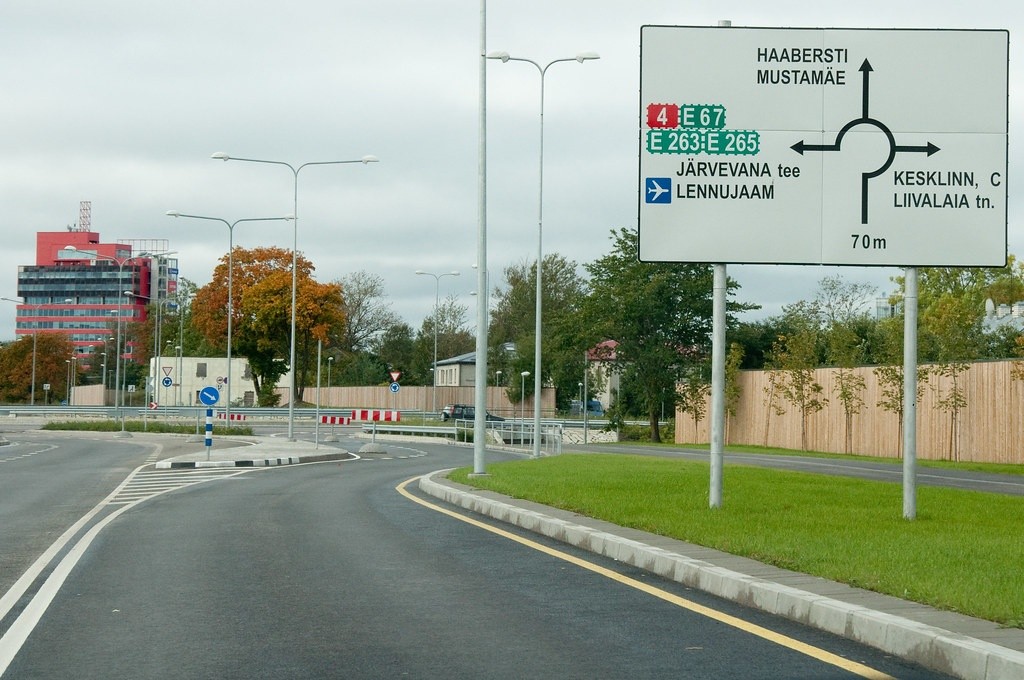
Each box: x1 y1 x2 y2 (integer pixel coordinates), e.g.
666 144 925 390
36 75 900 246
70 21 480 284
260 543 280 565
639 23 1008 267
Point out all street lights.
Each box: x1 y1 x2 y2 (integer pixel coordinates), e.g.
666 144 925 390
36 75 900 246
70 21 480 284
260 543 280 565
328 357 333 409
124 291 158 403
578 383 583 416
1 297 73 405
487 51 602 458
496 370 502 417
470 291 478 317
175 346 181 406
98 337 114 384
472 264 488 326
157 293 197 407
212 151 380 442
165 210 299 427
65 356 76 405
64 245 178 425
416 270 461 411
521 372 531 449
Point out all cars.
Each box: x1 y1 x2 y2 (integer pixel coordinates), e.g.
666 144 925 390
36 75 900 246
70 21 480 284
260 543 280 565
579 401 606 417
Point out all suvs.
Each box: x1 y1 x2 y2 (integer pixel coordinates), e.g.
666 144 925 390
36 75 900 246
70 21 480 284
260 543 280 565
440 403 505 428
